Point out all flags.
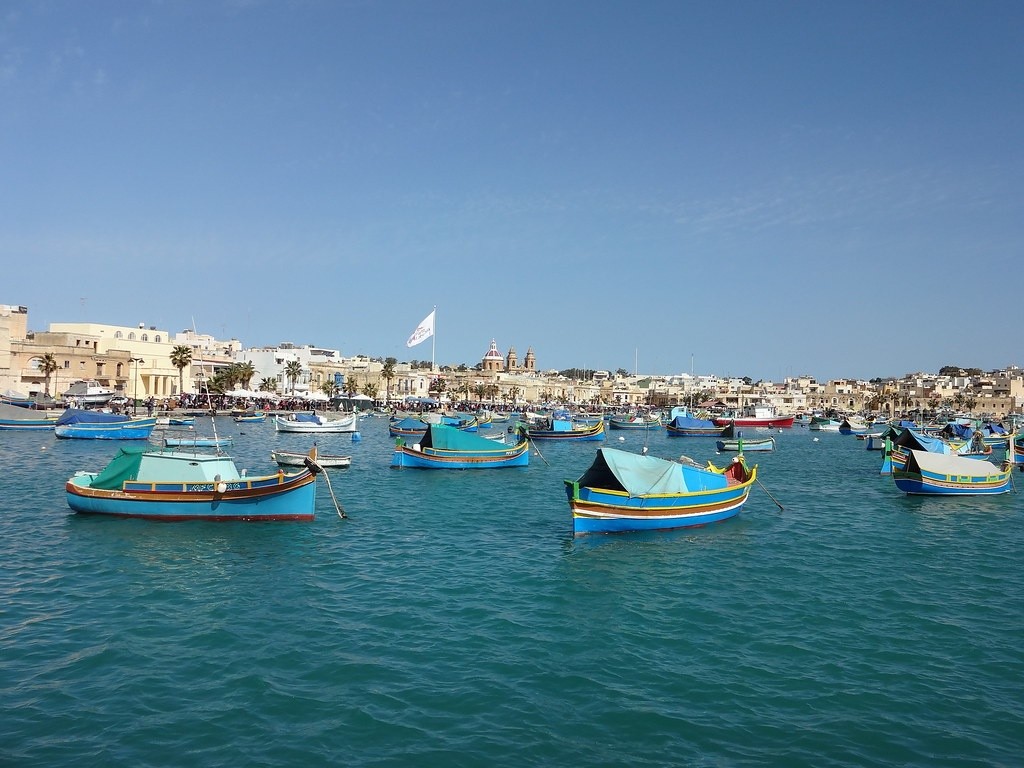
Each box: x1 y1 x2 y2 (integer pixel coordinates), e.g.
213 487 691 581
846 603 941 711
407 311 434 348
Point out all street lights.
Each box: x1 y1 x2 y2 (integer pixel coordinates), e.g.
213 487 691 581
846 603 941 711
127 357 146 414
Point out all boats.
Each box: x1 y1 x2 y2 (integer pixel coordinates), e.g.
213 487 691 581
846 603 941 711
796 411 1024 474
389 422 529 466
666 416 734 438
563 445 755 535
165 437 234 449
878 436 1013 496
61 379 115 407
234 414 267 422
515 409 606 441
274 407 358 433
709 416 793 427
54 407 157 439
716 438 774 450
132 415 197 426
607 403 731 430
0 402 56 430
359 407 513 436
271 448 353 468
0 392 36 410
64 315 318 522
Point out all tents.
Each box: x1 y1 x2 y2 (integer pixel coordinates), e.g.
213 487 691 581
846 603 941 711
225 388 374 402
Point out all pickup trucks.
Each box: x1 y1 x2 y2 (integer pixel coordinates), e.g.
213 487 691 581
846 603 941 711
28 391 57 410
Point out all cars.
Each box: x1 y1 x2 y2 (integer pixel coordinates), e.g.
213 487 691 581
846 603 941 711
109 396 128 407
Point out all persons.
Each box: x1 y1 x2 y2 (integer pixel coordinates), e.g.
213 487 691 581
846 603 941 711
372 401 533 413
181 394 312 411
